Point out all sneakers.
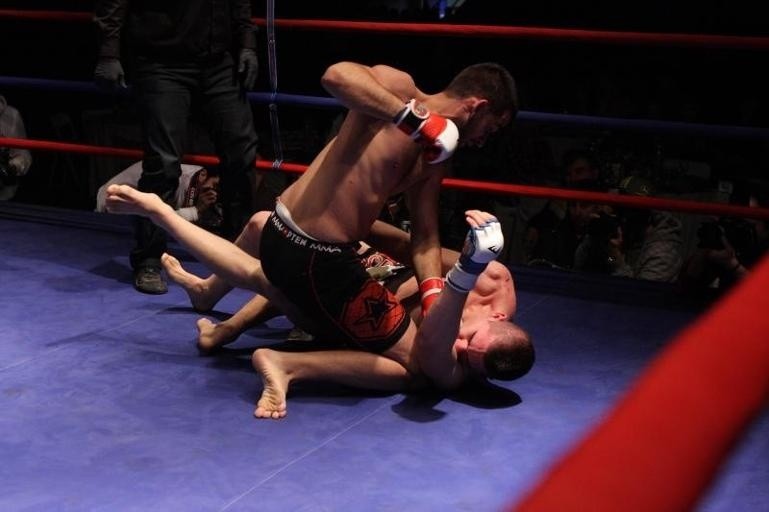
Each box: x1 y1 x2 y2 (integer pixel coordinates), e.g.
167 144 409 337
133 266 170 294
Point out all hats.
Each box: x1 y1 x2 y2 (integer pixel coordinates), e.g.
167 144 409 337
611 174 658 198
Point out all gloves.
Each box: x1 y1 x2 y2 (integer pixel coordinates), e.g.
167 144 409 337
393 99 461 165
444 217 506 292
237 47 260 91
92 47 129 91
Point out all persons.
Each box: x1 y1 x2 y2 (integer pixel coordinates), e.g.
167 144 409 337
483 141 769 292
90 0 269 297
102 183 537 383
191 57 519 420
1 92 33 203
